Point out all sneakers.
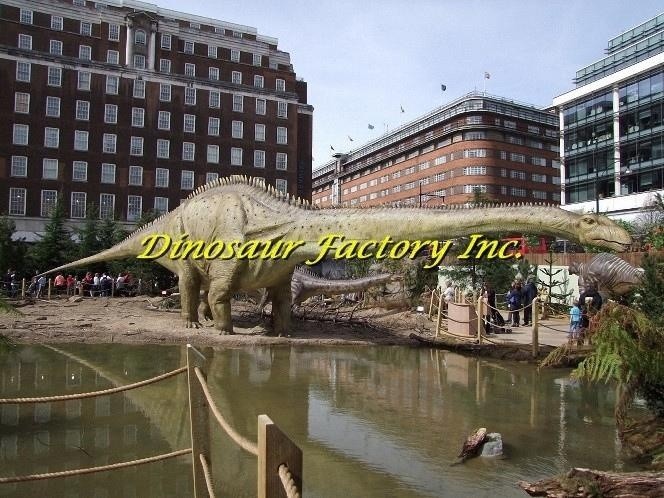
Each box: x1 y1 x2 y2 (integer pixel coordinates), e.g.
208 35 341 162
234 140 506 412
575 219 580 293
505 317 532 328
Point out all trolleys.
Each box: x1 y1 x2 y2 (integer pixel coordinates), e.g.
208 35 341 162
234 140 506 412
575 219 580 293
489 300 512 334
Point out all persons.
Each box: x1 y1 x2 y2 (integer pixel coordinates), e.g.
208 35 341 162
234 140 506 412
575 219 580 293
566 301 581 339
520 277 537 327
503 281 516 324
577 309 590 346
0 267 135 300
506 284 523 328
440 279 496 337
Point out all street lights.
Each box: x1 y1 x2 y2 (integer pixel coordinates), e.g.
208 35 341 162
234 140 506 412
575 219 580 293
593 136 632 215
417 183 447 210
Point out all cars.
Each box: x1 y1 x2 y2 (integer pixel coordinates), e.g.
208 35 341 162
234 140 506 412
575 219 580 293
430 230 586 254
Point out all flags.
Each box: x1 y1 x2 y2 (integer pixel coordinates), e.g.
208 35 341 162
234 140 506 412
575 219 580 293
311 68 491 162
